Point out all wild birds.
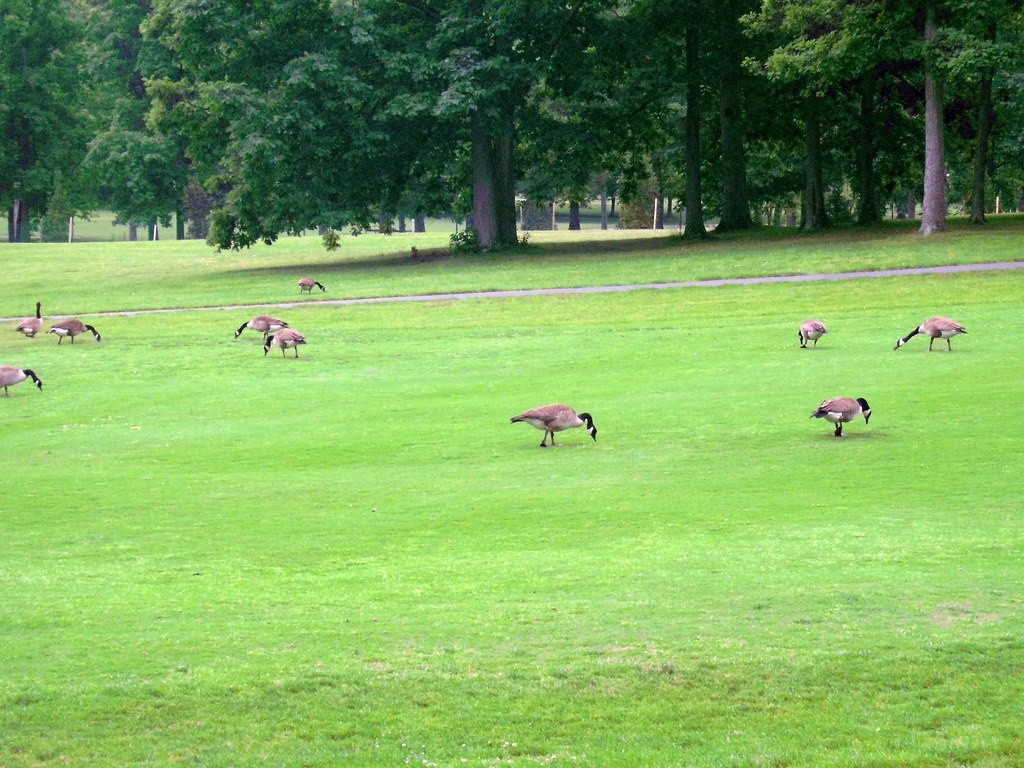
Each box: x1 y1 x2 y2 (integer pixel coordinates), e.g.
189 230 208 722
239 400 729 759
15 300 43 339
893 316 968 352
297 276 326 294
234 314 308 359
808 396 871 437
0 365 43 397
44 318 101 346
510 403 597 448
796 319 830 350
